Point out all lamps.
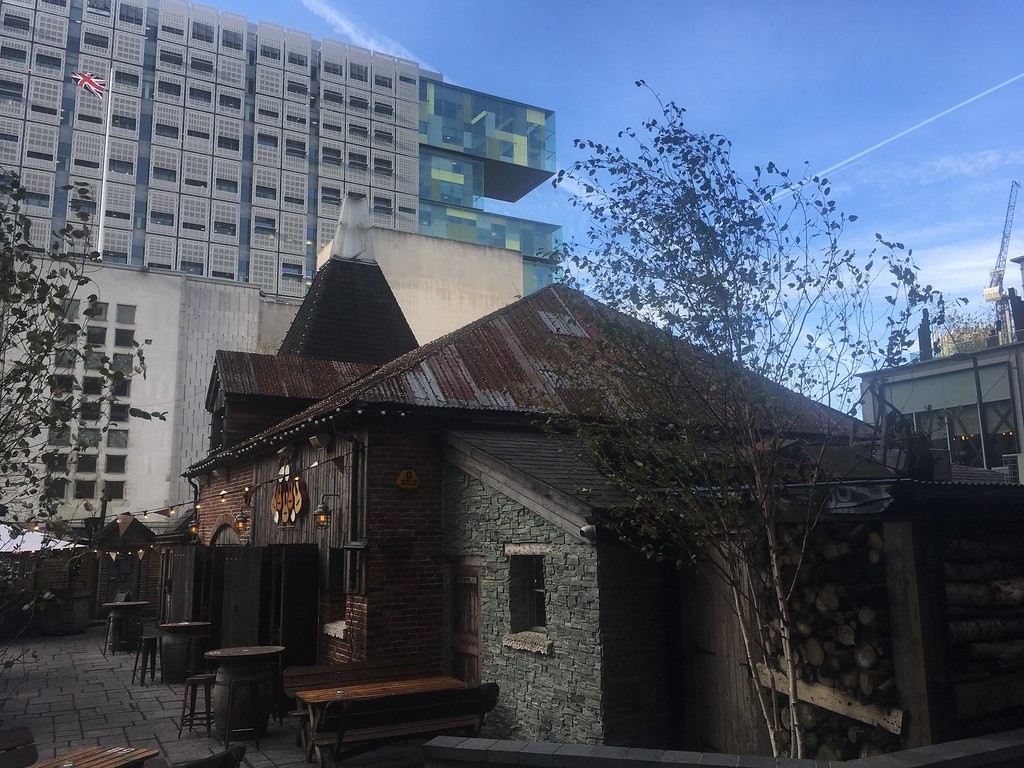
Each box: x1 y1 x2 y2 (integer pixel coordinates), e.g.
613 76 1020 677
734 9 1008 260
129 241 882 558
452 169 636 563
313 492 341 531
189 520 202 538
235 506 252 531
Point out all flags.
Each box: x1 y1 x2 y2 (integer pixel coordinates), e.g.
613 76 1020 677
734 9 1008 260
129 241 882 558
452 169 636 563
70 71 106 99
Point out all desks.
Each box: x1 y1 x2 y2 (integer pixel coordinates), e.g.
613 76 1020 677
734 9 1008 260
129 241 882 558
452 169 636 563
103 601 153 648
24 743 161 768
160 622 213 686
305 676 474 768
204 644 288 741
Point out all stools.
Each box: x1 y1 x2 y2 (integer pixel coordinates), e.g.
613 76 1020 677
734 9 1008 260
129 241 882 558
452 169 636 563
178 674 217 739
223 678 260 751
103 615 124 658
131 636 156 687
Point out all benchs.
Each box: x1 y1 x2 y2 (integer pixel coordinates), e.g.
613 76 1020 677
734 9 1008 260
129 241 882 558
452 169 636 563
287 710 320 717
169 743 247 768
284 657 434 753
0 725 38 768
310 713 475 748
